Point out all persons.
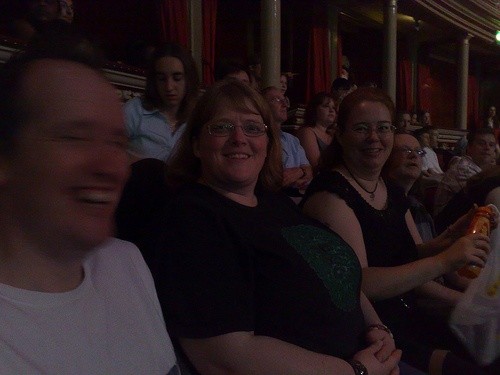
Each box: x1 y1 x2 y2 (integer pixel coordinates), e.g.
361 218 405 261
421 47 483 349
0 47 186 375
0 0 95 65
122 43 500 375
117 77 401 374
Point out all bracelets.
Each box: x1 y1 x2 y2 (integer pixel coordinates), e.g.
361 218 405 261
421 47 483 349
346 359 368 375
366 324 392 337
300 166 307 179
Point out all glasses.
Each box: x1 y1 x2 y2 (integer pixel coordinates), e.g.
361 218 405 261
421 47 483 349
206 119 269 137
271 96 290 105
351 123 398 137
392 148 427 157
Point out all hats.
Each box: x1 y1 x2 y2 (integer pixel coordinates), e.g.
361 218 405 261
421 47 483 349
332 78 351 90
343 64 353 76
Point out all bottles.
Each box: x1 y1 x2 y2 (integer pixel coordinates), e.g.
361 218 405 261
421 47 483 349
457 206 492 280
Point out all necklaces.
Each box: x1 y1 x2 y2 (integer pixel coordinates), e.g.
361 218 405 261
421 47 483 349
341 160 377 198
